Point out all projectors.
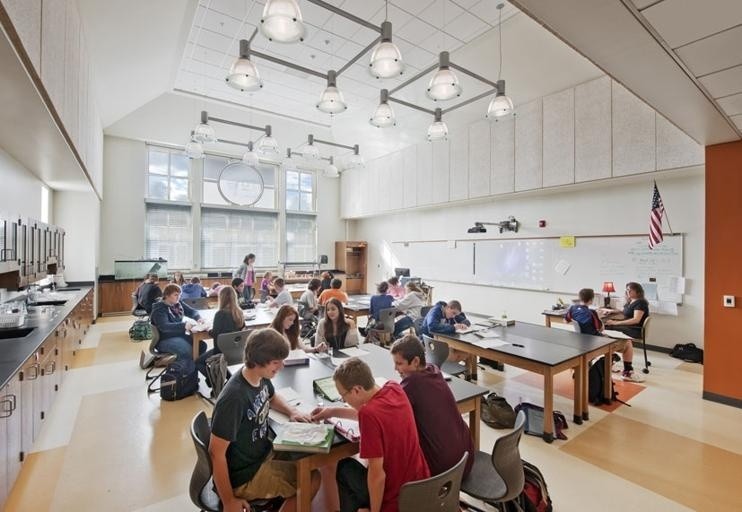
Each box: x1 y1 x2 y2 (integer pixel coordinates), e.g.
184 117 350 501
467 226 488 233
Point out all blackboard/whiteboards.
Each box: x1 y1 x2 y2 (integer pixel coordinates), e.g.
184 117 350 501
390 232 685 305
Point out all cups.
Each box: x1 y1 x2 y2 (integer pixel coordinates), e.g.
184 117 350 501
316 393 324 406
327 347 334 356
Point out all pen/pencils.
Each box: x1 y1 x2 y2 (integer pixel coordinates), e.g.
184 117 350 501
512 343 524 347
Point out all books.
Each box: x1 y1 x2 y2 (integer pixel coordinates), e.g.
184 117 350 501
313 376 342 402
327 417 360 444
544 305 574 315
272 421 334 455
242 309 256 320
454 314 515 334
283 349 309 366
268 386 316 425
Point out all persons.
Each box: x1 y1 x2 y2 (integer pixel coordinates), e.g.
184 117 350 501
131 251 290 362
391 332 476 486
561 287 621 379
206 326 322 512
299 269 471 362
310 353 433 512
601 281 649 340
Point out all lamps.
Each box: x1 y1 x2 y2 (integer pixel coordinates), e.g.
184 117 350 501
486 5 516 118
316 70 347 115
371 0 404 76
426 0 465 99
223 37 265 94
370 90 395 129
285 132 363 177
182 110 281 164
426 107 449 143
261 0 311 48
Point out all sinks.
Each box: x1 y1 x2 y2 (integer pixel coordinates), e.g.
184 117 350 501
57 288 83 292
0 326 39 341
30 299 68 307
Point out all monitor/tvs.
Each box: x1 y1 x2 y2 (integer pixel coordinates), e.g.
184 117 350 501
395 267 410 276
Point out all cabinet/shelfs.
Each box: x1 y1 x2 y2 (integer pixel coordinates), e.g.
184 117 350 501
1 283 93 510
1 0 105 200
334 242 368 295
0 215 66 288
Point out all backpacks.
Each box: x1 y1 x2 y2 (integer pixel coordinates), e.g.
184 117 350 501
588 354 620 406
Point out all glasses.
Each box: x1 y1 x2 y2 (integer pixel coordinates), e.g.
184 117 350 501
337 390 351 402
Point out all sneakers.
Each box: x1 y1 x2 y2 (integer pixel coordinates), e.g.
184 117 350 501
621 370 645 382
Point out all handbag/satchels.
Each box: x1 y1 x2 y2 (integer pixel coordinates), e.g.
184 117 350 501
161 359 199 401
480 392 515 428
669 343 703 364
514 403 568 440
499 459 552 511
129 321 153 339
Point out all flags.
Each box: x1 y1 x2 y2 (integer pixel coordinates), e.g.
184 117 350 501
648 179 664 250
